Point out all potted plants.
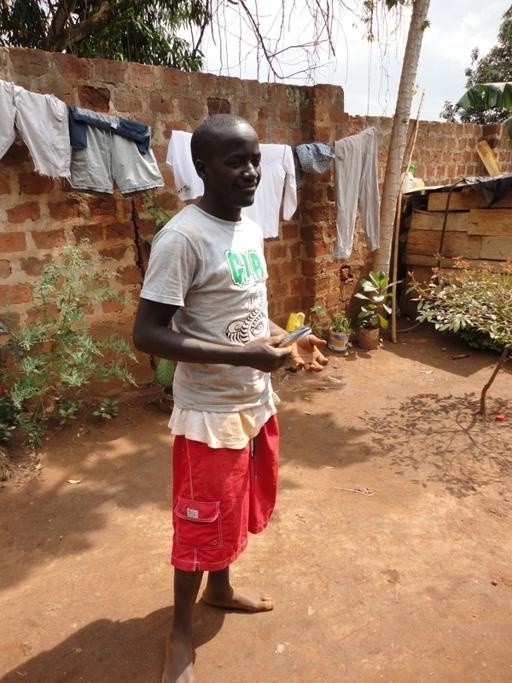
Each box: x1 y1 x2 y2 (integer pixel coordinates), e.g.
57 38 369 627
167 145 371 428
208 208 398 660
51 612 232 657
355 269 405 351
327 312 353 352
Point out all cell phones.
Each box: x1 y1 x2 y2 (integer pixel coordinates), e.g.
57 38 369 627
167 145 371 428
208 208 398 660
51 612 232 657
276 325 312 348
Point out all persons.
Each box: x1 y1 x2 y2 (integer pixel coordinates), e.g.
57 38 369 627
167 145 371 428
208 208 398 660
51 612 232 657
131 112 330 683
